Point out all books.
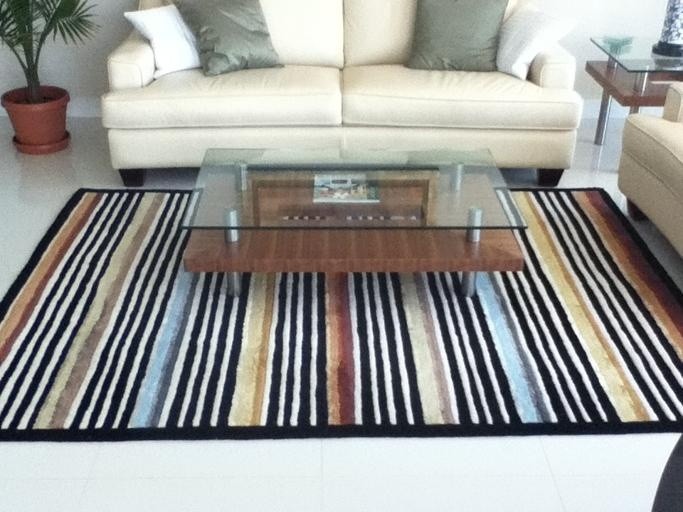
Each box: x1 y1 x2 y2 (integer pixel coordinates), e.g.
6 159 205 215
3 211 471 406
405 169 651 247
311 170 380 206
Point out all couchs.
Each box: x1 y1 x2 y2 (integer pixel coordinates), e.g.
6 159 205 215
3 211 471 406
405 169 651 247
98 2 586 197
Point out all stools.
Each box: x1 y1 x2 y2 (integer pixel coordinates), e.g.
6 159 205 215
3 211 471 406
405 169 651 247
617 80 683 266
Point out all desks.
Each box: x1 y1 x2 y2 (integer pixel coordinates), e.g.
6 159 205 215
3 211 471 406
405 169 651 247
586 28 683 149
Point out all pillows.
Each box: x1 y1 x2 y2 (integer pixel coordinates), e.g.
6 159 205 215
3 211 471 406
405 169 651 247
495 2 578 82
342 3 420 70
261 2 345 71
403 2 508 72
174 2 285 78
122 4 203 81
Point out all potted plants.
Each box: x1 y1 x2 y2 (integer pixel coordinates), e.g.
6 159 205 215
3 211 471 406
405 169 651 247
0 1 102 145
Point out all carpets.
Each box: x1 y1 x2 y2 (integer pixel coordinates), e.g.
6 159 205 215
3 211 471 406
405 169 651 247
1 186 683 443
